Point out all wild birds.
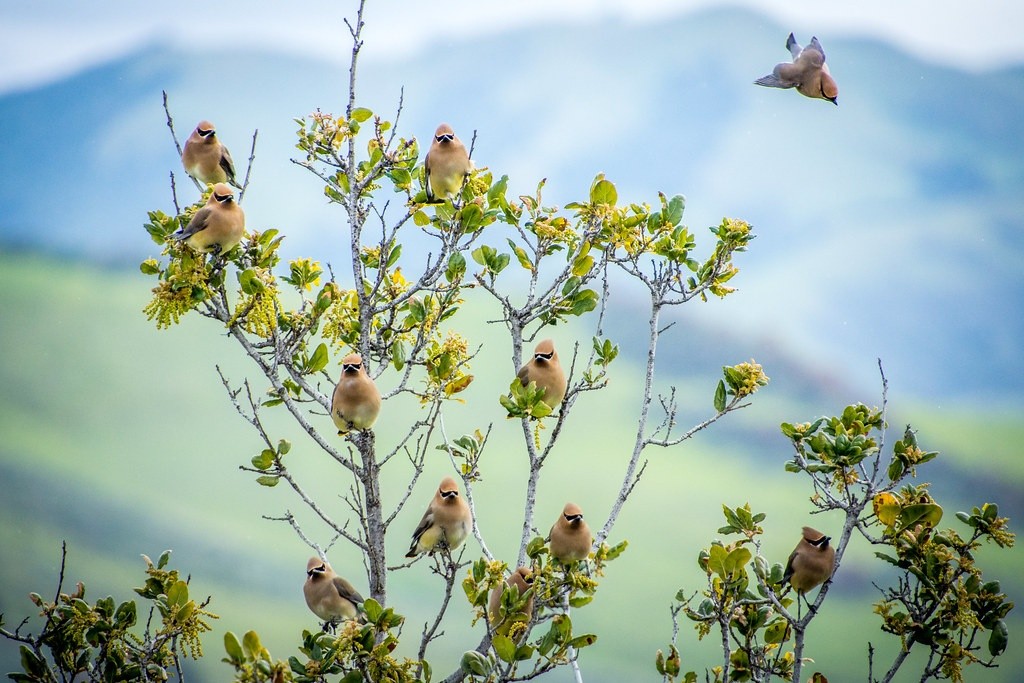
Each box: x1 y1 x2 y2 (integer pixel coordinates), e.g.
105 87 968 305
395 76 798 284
774 525 840 617
403 476 476 574
542 499 597 566
512 337 570 421
328 351 388 441
300 557 371 637
488 567 536 652
166 183 253 264
418 120 476 204
753 32 843 108
170 121 271 192
